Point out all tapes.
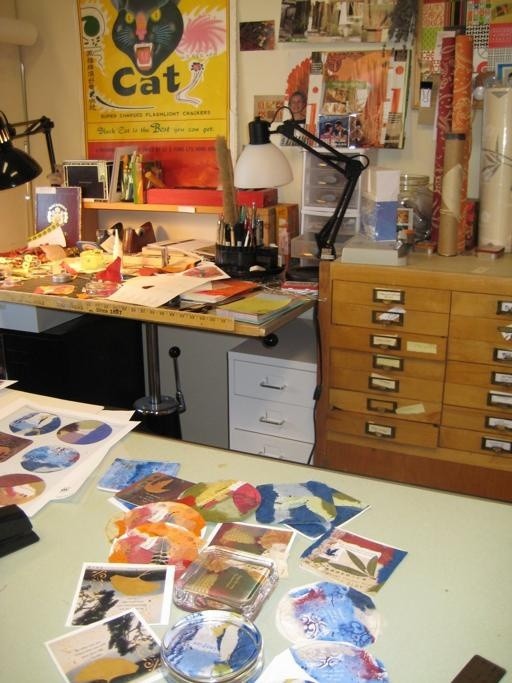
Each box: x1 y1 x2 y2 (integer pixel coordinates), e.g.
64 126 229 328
135 227 145 236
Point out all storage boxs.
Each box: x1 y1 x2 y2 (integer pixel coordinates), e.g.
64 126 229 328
299 146 364 245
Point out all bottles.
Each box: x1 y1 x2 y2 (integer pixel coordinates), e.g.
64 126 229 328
397 175 434 242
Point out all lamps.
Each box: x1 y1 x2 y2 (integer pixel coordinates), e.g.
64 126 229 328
233 110 369 258
0 105 58 192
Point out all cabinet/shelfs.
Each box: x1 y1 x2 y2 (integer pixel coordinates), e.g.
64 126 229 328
305 254 512 504
225 315 320 468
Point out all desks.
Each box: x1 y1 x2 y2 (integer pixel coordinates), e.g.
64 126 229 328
1 398 512 683
0 253 314 424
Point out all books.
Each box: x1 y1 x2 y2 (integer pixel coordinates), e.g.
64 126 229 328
60 144 165 203
34 185 82 252
121 235 216 275
274 202 300 268
253 206 277 249
217 291 313 322
178 276 260 304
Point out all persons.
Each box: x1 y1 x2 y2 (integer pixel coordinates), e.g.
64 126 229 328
319 120 335 147
288 91 306 122
332 119 350 146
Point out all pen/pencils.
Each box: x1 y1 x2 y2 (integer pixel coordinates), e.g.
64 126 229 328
217 202 264 248
